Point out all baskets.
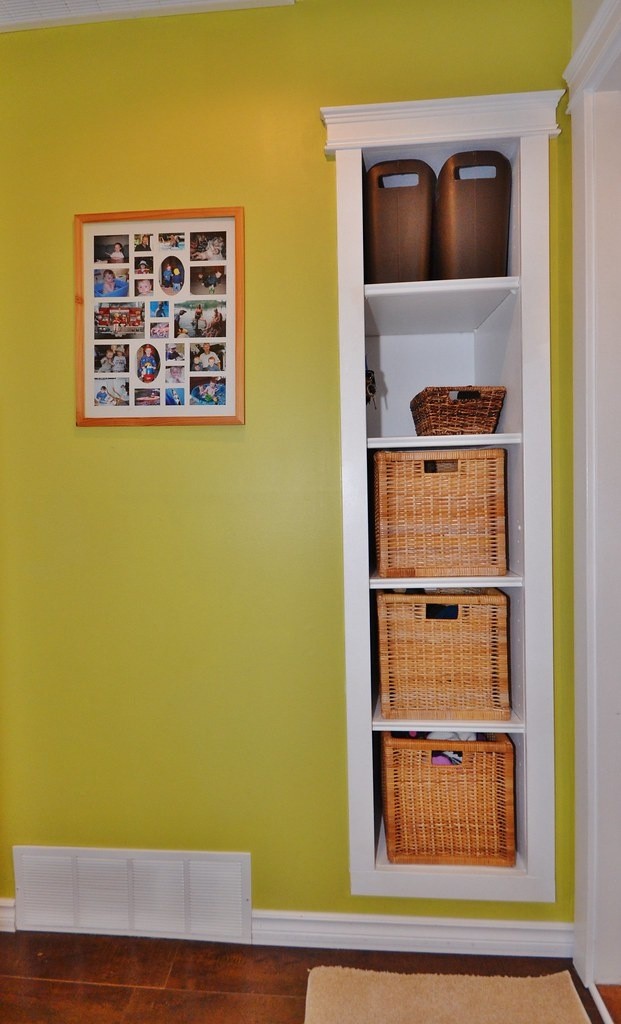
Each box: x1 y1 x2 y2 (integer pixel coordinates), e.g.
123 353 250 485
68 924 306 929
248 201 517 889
411 386 507 435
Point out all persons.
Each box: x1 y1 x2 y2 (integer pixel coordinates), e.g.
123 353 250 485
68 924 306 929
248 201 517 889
96 236 225 405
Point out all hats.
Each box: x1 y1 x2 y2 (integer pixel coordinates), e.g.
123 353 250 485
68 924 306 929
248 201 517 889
144 345 155 354
115 347 125 353
139 261 148 266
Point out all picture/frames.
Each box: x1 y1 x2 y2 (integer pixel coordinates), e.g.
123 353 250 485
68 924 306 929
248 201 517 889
71 204 248 428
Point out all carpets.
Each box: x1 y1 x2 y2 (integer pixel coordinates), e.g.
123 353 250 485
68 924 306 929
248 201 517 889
301 966 596 1024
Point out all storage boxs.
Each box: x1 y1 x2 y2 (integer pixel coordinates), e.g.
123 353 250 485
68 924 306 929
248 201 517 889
368 159 435 280
436 150 514 276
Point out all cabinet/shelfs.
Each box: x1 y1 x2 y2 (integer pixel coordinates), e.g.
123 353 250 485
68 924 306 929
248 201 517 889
318 87 567 900
